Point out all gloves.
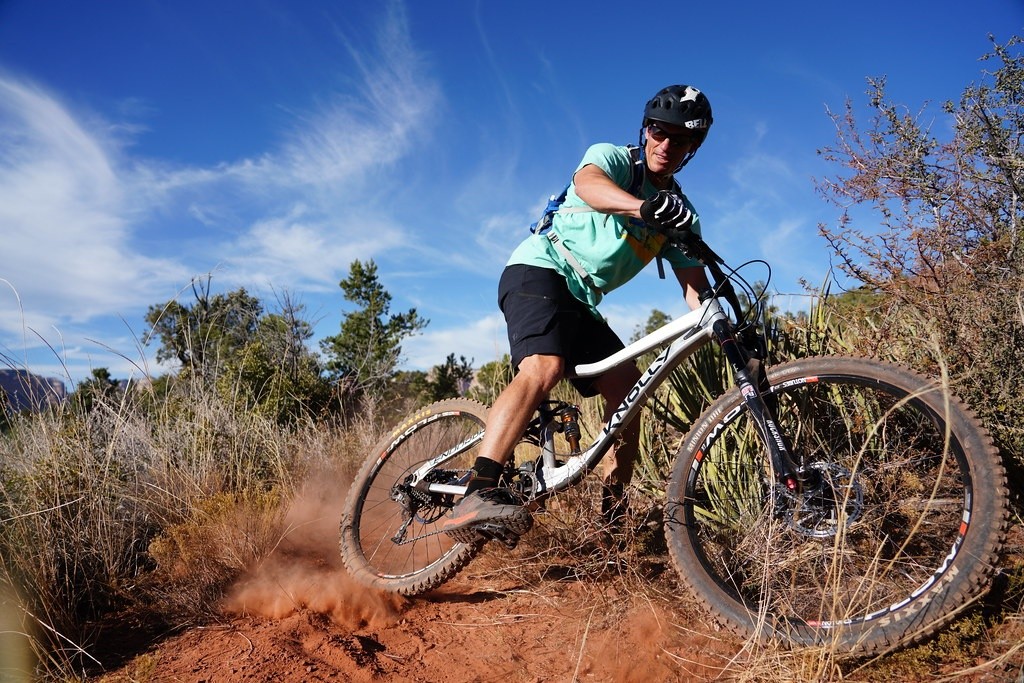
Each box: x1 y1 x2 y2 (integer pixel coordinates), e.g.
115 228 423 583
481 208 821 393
640 190 693 237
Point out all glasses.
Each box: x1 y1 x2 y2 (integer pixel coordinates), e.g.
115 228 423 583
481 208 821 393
648 122 698 148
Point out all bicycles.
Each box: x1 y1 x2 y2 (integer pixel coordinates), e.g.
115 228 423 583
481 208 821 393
336 227 1009 664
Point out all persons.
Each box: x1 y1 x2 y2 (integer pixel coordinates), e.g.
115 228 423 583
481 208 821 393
442 85 768 544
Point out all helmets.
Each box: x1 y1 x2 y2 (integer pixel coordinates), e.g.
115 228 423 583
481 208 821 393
643 84 713 149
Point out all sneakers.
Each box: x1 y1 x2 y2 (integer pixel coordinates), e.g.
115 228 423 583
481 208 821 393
443 486 534 544
604 554 665 580
